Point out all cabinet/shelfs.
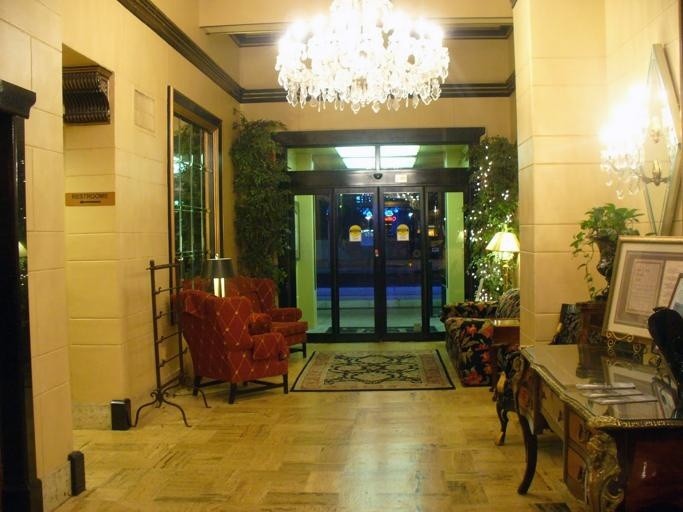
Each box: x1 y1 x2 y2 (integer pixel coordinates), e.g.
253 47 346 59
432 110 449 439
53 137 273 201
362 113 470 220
495 347 683 512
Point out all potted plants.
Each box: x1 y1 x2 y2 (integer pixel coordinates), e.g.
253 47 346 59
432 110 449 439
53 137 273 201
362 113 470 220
569 201 656 301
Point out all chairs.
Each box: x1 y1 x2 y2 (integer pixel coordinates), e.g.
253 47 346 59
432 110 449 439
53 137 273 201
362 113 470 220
169 274 309 404
439 288 520 387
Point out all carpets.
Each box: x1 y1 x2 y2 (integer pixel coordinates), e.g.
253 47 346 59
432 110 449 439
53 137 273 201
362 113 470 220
290 348 456 392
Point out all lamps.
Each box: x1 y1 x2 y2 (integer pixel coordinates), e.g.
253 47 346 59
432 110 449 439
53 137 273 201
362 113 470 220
272 0 452 116
485 231 521 293
207 258 233 299
